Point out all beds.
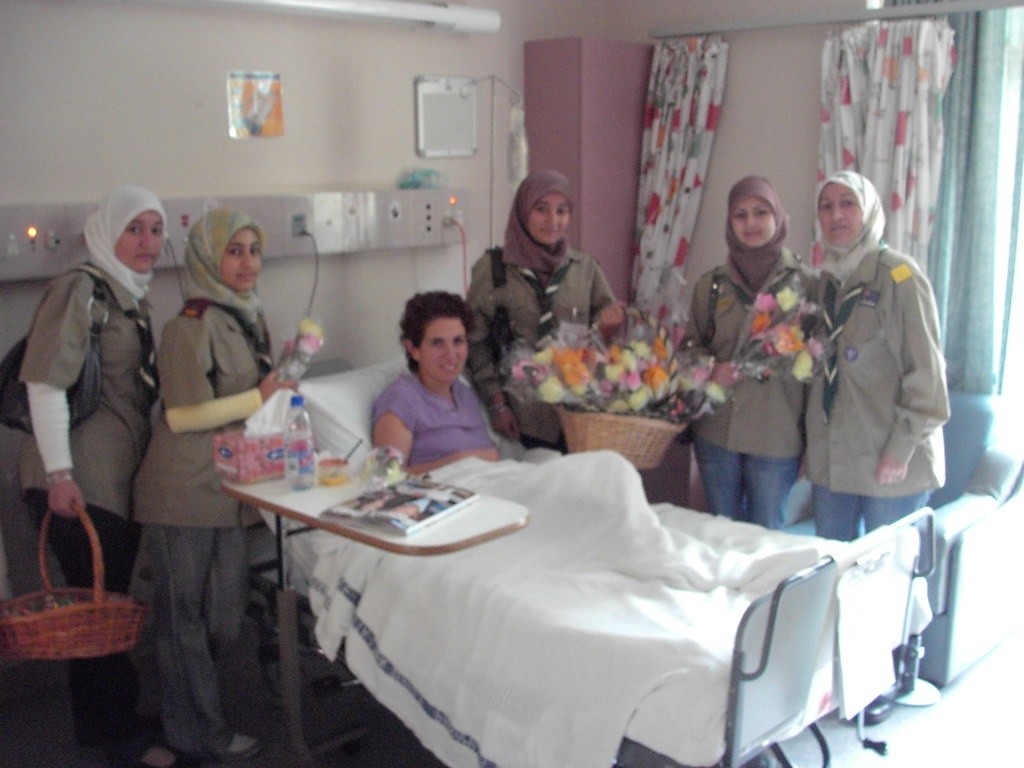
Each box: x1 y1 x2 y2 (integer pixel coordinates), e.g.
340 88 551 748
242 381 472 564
244 362 938 768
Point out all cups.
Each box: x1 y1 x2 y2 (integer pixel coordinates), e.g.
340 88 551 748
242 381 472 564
315 459 349 486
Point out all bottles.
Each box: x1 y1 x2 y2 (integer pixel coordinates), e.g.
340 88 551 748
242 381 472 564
283 395 317 491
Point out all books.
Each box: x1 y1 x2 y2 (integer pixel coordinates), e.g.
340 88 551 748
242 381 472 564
324 478 478 536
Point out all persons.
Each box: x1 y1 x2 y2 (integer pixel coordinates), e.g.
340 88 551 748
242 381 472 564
18 182 298 768
464 167 626 453
373 290 501 477
680 172 951 724
354 483 454 518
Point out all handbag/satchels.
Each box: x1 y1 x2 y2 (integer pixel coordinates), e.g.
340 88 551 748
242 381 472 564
1 268 110 431
484 247 512 361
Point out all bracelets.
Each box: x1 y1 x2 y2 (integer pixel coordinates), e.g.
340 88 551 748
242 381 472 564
46 472 72 489
488 401 505 414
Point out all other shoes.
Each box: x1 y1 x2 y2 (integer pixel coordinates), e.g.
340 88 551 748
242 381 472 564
863 680 896 724
227 733 265 761
107 741 199 768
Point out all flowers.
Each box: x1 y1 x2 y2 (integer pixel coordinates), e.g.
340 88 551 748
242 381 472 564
500 304 734 423
730 286 827 384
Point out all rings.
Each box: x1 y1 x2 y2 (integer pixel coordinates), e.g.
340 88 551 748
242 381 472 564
609 311 616 317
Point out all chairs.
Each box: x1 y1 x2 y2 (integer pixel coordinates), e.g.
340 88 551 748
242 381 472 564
783 392 1024 688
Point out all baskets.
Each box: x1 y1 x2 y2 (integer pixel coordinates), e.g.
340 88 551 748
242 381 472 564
554 307 688 472
0 499 155 662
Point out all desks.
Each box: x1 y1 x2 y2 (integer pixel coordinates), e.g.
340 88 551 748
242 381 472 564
222 467 529 762
